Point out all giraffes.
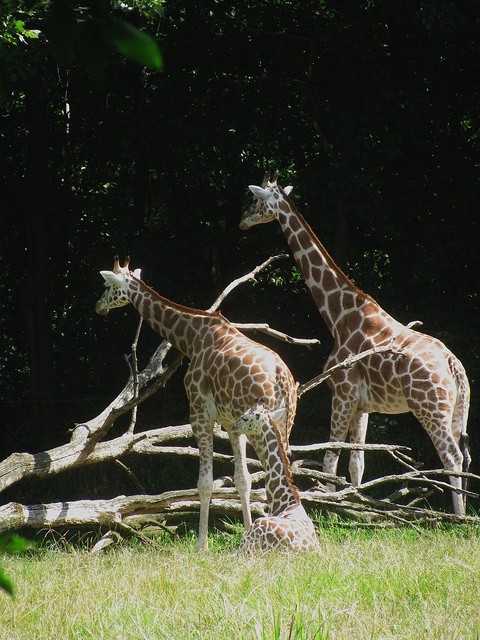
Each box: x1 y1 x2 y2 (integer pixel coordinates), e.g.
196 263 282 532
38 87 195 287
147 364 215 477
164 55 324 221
238 167 472 519
93 255 300 552
231 397 325 560
